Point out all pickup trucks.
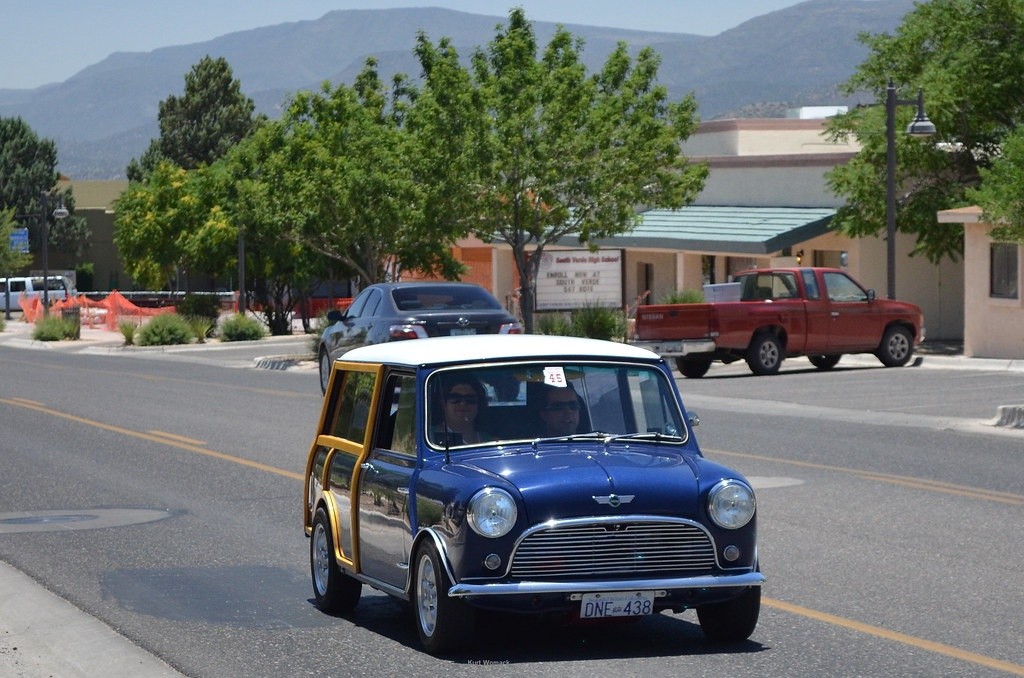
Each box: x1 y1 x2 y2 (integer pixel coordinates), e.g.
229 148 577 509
632 266 927 378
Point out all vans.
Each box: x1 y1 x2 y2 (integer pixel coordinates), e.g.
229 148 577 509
0 275 78 311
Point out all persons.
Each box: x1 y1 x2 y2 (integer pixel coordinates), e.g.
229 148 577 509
427 375 501 449
534 380 581 438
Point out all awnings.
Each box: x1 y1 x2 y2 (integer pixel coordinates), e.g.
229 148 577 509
479 204 842 255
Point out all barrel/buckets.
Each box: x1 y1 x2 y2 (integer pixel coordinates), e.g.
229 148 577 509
703 282 741 303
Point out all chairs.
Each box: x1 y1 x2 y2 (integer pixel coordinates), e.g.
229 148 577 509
806 283 814 297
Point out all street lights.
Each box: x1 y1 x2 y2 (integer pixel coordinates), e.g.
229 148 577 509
40 183 68 318
885 75 938 300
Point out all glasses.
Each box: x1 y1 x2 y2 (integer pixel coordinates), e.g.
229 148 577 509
543 400 581 412
446 392 480 405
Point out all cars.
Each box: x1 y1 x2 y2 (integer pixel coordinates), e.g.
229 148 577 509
319 282 527 404
303 331 768 656
